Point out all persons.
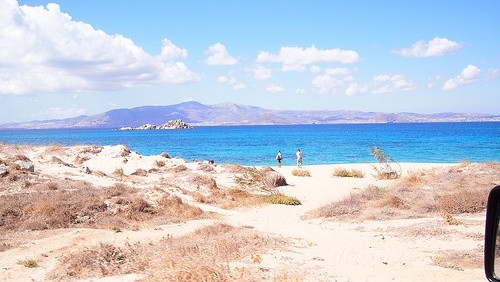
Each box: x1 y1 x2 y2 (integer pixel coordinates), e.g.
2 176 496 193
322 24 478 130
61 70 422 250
295 149 303 167
193 158 214 165
277 151 282 167
